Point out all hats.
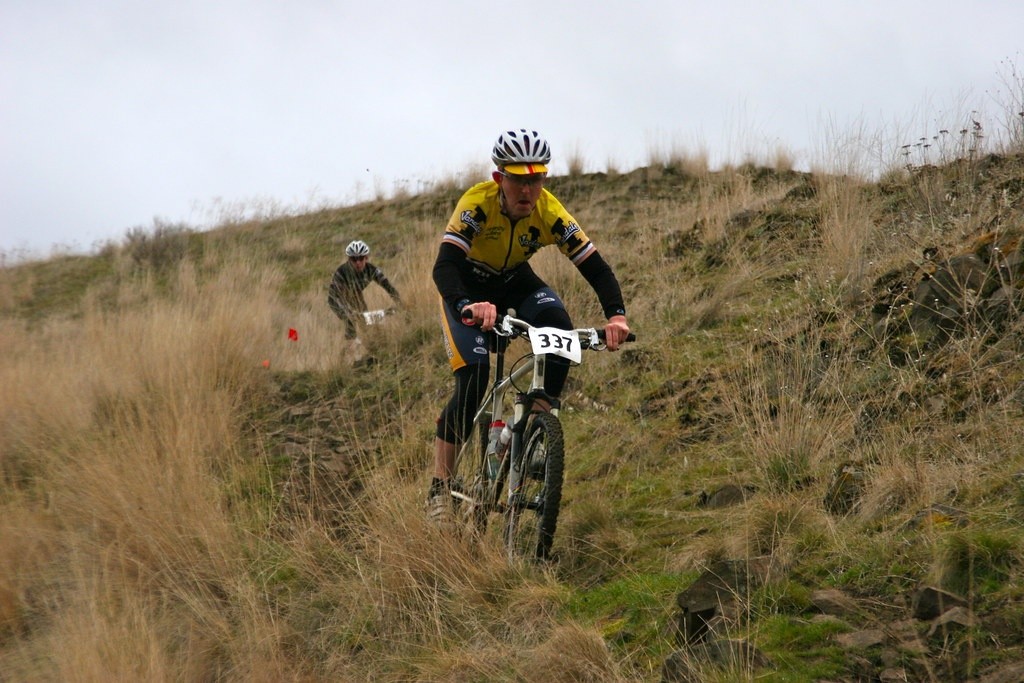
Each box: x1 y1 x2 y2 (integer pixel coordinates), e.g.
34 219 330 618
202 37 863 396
498 162 549 174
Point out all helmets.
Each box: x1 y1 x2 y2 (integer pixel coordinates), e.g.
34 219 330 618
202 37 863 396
345 241 370 256
491 129 551 165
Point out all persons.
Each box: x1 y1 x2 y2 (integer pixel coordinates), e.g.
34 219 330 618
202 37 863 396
328 239 409 366
425 127 630 529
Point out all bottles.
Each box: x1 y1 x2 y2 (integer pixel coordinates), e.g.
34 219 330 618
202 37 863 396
487 419 505 480
494 415 514 462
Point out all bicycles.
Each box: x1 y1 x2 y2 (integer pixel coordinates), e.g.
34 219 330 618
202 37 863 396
342 305 400 339
445 306 636 561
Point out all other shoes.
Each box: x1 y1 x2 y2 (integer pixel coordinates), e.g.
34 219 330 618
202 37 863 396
422 492 455 533
527 442 547 481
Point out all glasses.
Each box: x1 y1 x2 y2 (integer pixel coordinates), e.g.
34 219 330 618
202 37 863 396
348 255 367 262
498 170 546 187
